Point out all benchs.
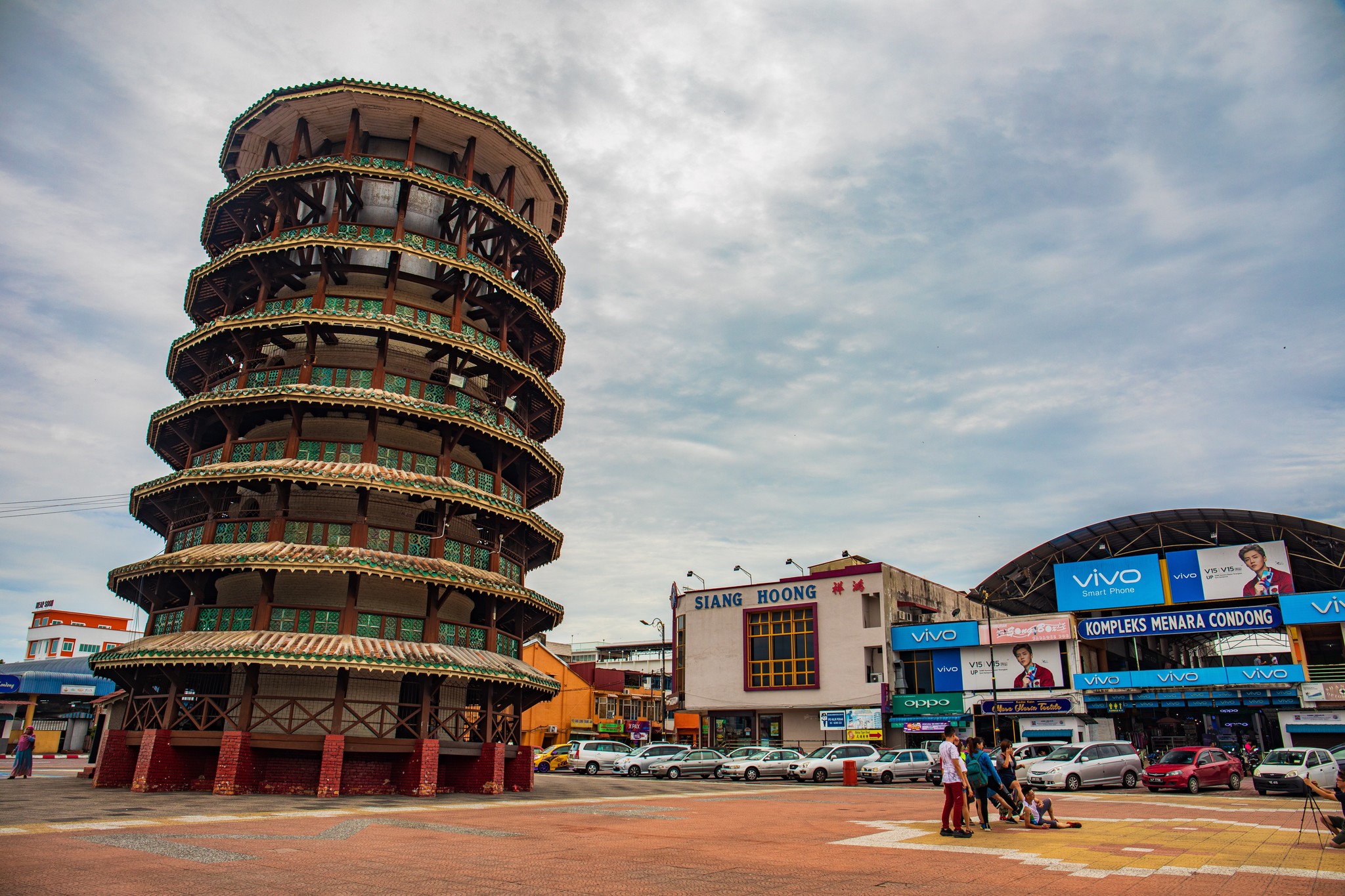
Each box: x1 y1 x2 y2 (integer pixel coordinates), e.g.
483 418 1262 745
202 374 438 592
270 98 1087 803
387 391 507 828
783 756 796 760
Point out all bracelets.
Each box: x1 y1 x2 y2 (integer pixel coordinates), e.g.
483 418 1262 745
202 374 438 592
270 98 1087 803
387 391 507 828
968 786 971 788
1006 754 1009 756
1042 825 1044 829
1020 764 1022 768
954 745 958 747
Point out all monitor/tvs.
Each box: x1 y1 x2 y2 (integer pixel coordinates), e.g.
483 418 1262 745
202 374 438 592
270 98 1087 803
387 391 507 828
449 373 466 389
503 395 517 413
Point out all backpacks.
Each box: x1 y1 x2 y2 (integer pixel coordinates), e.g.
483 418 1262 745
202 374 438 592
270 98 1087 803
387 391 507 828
994 755 1015 774
966 751 988 788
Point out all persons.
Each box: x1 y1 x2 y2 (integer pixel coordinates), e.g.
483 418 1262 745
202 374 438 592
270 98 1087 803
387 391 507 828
854 717 873 728
958 741 975 825
1207 725 1219 735
965 737 1018 825
1304 770 1345 848
996 740 1025 824
1013 643 1055 688
1209 742 1214 747
1270 653 1278 666
1235 727 1244 752
7 725 36 779
1245 739 1256 753
975 788 1016 829
965 737 1025 831
953 741 974 834
1036 750 1046 757
940 736 966 826
1021 786 1082 829
998 771 1026 822
939 725 972 838
1238 544 1294 597
1254 655 1266 666
976 796 1001 824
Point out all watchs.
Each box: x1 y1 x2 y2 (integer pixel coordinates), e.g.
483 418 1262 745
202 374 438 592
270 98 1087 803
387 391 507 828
1041 802 1044 806
1317 785 1319 788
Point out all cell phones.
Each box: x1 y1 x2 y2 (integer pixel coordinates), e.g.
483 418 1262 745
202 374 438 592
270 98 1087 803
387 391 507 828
1260 570 1273 590
1307 772 1309 778
1027 666 1037 683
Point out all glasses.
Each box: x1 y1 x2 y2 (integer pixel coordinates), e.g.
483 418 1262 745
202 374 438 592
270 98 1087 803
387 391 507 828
1336 779 1345 782
1026 791 1035 796
1008 746 1012 749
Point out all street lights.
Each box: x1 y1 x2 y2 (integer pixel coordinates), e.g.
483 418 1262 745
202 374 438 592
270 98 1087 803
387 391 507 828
622 650 624 661
572 634 573 652
649 648 651 660
969 585 1000 745
639 618 665 742
635 650 637 661
609 652 611 662
603 639 605 645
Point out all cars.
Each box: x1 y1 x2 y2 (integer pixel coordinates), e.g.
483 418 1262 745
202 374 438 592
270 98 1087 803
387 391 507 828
720 749 806 782
1327 743 1345 772
781 746 808 757
705 746 731 756
532 744 572 773
725 746 778 761
1252 747 1340 797
860 740 997 786
1141 746 1244 794
647 749 734 780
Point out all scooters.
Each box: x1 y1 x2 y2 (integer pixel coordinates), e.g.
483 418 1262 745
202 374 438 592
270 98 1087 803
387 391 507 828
1227 745 1262 777
1144 750 1161 765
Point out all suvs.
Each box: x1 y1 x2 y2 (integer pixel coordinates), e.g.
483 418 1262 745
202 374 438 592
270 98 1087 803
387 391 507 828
787 743 881 783
1026 740 1142 792
988 740 1068 785
567 740 635 775
612 744 693 777
644 741 670 752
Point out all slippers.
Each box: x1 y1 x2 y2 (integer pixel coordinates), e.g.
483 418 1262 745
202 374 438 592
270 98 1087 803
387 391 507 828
969 819 975 825
1012 787 1018 803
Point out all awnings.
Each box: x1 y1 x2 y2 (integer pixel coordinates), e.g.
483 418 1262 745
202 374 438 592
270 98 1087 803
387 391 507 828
889 714 973 723
1286 724 1345 733
0 700 38 705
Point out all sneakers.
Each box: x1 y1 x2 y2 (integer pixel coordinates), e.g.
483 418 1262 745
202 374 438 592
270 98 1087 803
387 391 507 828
1066 821 1082 828
984 823 991 831
1015 802 1025 816
940 827 953 836
952 828 972 838
1051 818 1059 823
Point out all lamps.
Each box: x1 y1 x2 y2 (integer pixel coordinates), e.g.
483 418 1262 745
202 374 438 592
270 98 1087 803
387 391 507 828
449 373 468 390
786 558 804 577
1099 543 1114 559
1210 532 1218 547
500 395 517 410
687 571 705 589
734 565 752 585
842 550 857 565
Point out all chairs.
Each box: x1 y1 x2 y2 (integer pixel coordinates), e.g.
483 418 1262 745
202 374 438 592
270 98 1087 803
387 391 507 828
1022 750 1031 760
769 756 775 760
691 755 696 760
607 746 612 751
603 746 607 751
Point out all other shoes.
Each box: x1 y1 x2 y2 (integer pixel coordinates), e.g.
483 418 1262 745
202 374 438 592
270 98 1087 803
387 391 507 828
980 823 992 830
1019 816 1025 822
999 816 1007 821
1005 817 1018 824
961 822 966 826
993 801 1001 810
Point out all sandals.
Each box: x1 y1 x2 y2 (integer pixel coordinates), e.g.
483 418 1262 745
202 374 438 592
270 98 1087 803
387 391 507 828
966 828 974 834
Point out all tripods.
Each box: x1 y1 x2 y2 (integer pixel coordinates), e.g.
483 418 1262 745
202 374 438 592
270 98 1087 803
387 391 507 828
1297 792 1335 849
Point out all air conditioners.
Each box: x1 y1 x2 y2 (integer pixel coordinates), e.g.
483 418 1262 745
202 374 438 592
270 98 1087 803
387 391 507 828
927 618 935 623
870 673 884 683
548 725 558 733
616 719 622 724
896 611 905 620
904 613 912 621
623 689 629 694
914 614 924 622
